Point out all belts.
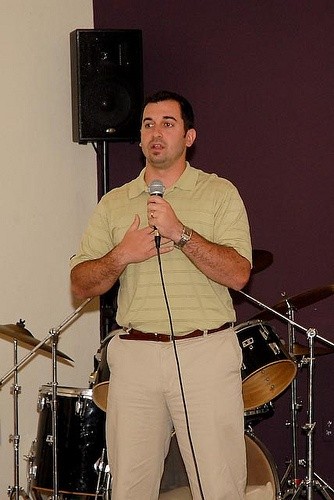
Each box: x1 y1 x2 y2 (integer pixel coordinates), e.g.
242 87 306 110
119 322 231 343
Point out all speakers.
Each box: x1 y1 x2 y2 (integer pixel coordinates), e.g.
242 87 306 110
69 28 143 145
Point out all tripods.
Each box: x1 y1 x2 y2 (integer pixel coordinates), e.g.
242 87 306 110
239 292 334 500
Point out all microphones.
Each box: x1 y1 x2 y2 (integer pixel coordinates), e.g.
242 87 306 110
149 180 164 249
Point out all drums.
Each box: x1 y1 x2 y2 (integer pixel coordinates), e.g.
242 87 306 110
92 329 126 412
30 384 108 496
159 422 282 500
233 319 297 411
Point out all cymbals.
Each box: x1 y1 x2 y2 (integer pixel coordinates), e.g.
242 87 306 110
283 342 334 355
252 249 273 273
0 323 74 362
246 285 334 321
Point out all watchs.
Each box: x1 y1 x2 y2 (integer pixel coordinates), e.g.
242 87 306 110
174 224 192 249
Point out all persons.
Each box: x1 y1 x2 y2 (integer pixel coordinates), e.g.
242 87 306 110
70 92 252 499
